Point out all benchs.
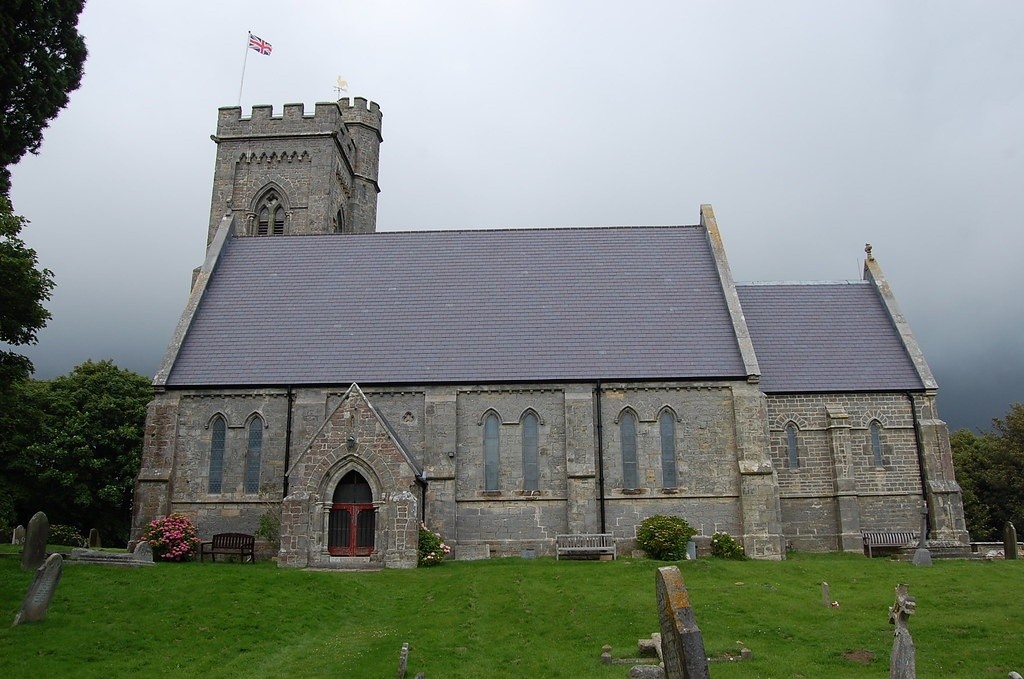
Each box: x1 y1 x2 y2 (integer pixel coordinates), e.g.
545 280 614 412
862 529 917 558
202 533 254 564
556 530 616 560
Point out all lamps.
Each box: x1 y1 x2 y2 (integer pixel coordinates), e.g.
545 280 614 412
449 451 454 457
348 434 356 447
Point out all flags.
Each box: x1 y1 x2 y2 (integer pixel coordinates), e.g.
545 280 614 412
249 35 272 56
